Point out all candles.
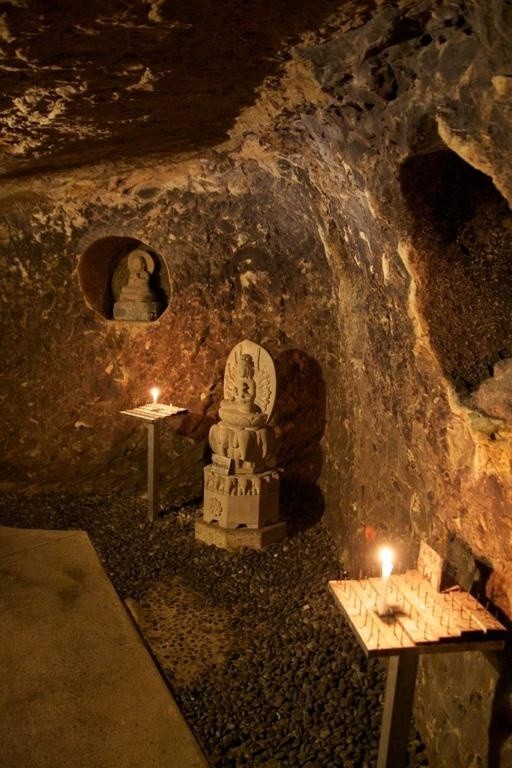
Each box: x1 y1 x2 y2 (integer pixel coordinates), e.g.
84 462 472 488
152 388 158 411
378 551 395 612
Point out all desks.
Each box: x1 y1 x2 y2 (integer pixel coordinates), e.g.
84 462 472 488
119 402 188 524
327 569 509 767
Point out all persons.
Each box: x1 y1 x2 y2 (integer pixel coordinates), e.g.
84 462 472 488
121 255 153 303
220 354 260 414
